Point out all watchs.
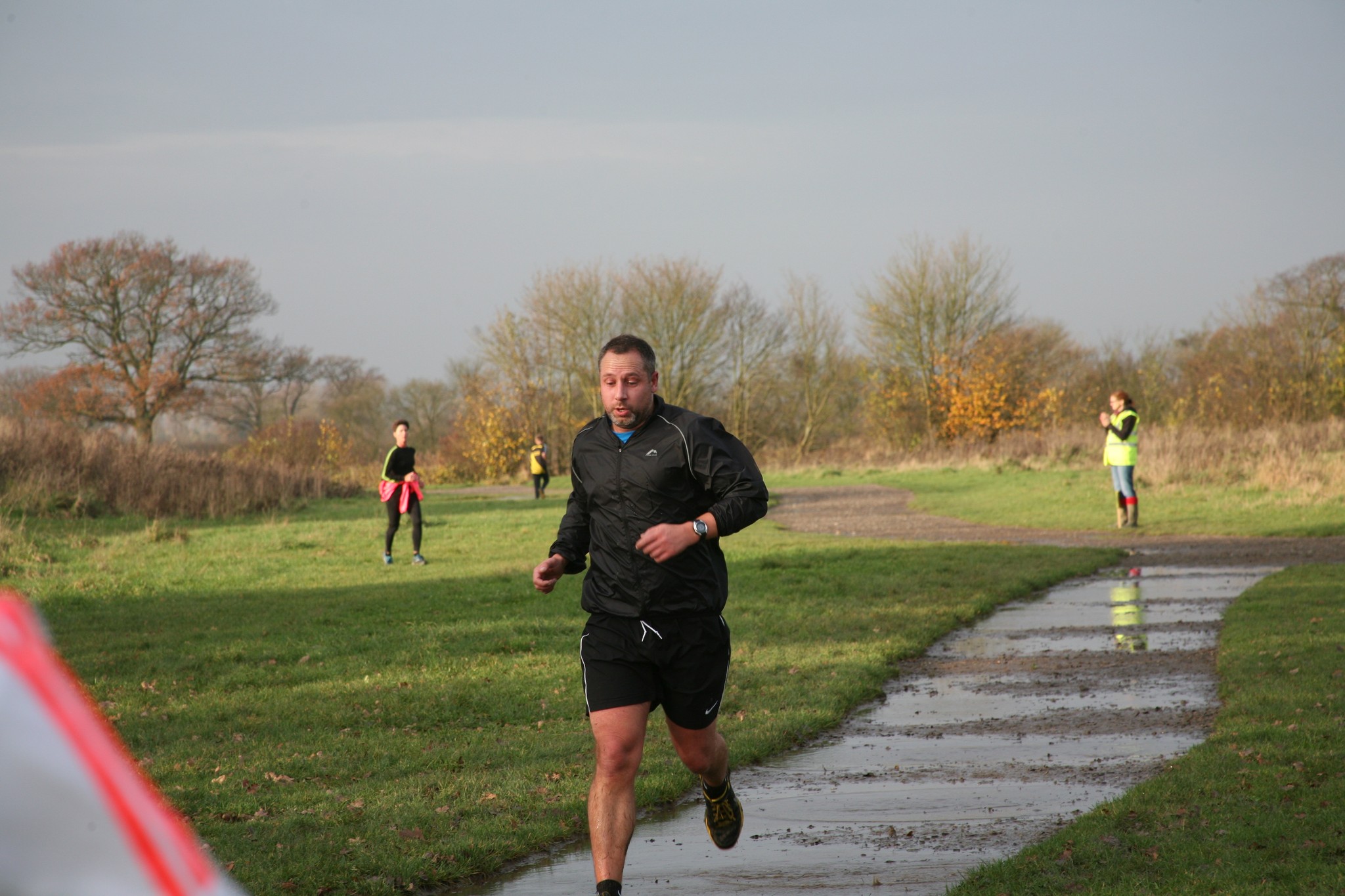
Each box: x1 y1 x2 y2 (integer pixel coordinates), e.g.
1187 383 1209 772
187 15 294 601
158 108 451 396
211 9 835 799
689 518 708 542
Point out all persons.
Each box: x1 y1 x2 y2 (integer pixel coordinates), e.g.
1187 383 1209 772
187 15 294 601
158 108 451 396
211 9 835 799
1099 391 1142 528
531 333 770 896
381 419 430 565
530 434 549 501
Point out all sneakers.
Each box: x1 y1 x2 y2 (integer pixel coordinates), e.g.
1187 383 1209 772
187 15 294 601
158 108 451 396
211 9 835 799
383 553 393 565
413 554 426 565
698 772 744 850
